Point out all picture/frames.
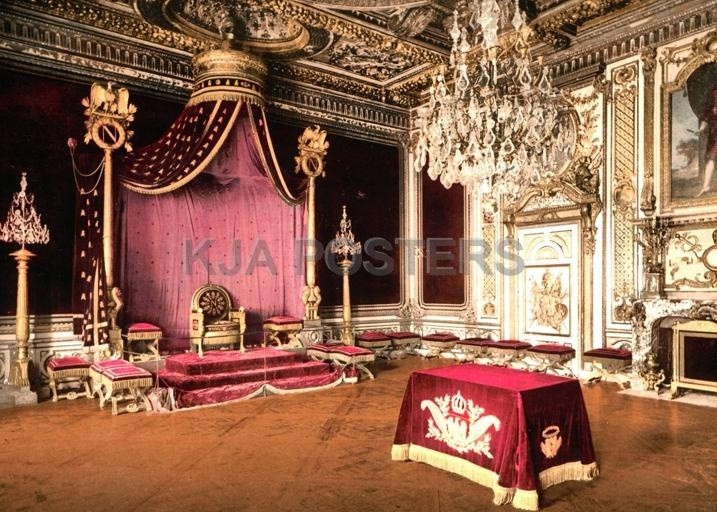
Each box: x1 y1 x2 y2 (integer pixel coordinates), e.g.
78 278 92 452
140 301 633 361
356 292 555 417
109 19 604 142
659 52 716 213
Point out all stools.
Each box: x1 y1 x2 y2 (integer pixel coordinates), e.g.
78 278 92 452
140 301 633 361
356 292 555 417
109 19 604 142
45 356 153 416
121 323 161 364
263 315 302 349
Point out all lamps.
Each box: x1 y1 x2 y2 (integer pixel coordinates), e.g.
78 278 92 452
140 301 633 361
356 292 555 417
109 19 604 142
412 1 555 196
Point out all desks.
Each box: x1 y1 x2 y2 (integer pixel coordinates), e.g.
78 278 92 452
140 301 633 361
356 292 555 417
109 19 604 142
411 364 591 509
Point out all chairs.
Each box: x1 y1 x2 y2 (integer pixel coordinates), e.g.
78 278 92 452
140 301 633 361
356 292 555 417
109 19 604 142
189 285 247 357
